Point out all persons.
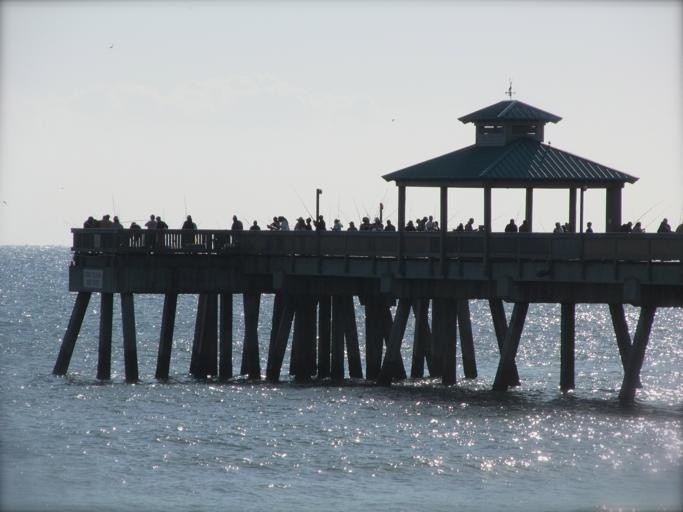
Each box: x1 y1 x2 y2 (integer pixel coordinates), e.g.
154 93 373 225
250 220 259 230
405 216 439 231
621 221 645 232
232 215 243 230
294 215 395 231
182 215 197 247
130 214 168 248
505 219 528 232
455 218 476 231
84 215 123 248
268 215 288 231
554 222 570 232
586 222 593 232
657 218 683 232
